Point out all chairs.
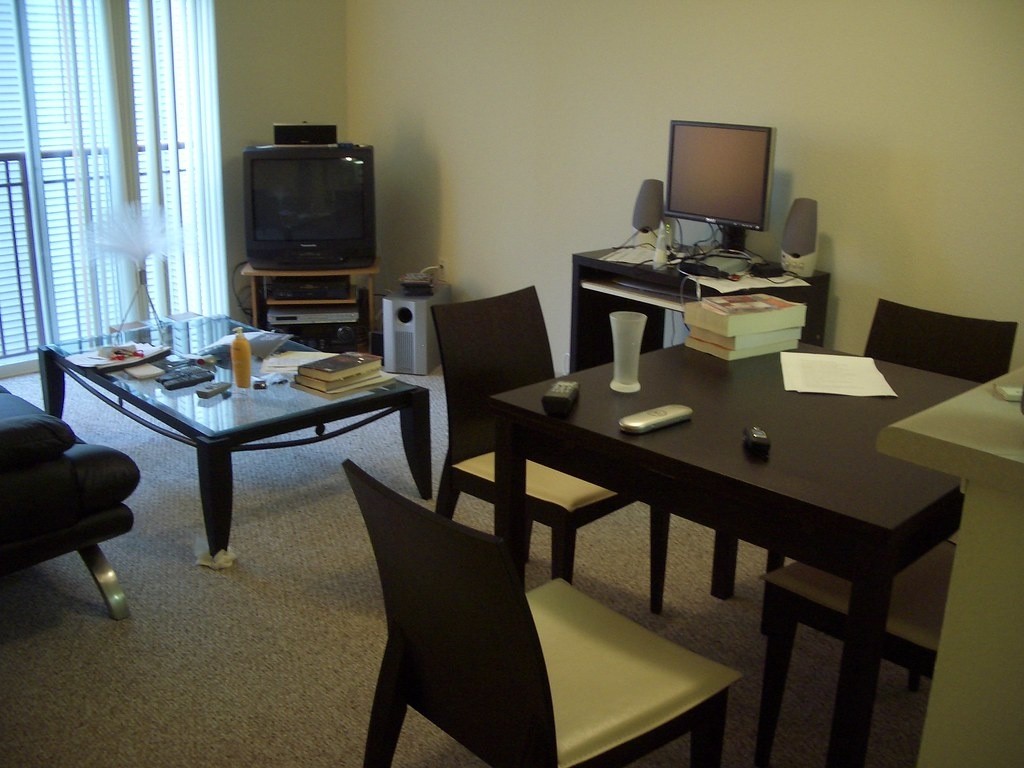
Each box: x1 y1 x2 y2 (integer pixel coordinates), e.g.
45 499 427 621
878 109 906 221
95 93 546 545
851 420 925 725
429 284 670 616
338 459 741 768
753 297 1017 763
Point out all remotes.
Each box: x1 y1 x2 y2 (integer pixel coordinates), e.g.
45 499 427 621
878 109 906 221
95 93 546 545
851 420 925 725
195 382 232 399
155 366 216 391
540 381 580 412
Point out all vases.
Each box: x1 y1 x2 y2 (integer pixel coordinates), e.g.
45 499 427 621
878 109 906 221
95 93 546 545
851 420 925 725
112 269 179 351
608 312 647 393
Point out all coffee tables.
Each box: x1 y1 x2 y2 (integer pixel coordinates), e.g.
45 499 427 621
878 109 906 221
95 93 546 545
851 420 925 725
37 314 433 562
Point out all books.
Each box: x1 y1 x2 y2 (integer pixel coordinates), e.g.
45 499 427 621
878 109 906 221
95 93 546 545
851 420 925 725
290 351 399 399
683 294 807 361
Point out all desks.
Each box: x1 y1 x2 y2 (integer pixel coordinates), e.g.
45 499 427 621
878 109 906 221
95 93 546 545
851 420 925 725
240 261 383 352
488 339 991 768
570 243 831 374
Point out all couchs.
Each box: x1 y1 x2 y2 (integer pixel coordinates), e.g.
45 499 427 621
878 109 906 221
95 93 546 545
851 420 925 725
0 388 142 622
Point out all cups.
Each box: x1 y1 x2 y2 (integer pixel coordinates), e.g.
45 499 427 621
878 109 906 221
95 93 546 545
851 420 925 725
609 312 647 393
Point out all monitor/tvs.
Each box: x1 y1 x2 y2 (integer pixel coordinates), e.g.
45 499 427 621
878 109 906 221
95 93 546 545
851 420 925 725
242 143 376 271
663 119 777 232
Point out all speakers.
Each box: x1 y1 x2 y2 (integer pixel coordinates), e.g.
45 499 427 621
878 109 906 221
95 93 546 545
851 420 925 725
631 179 664 251
780 197 819 277
381 281 451 376
